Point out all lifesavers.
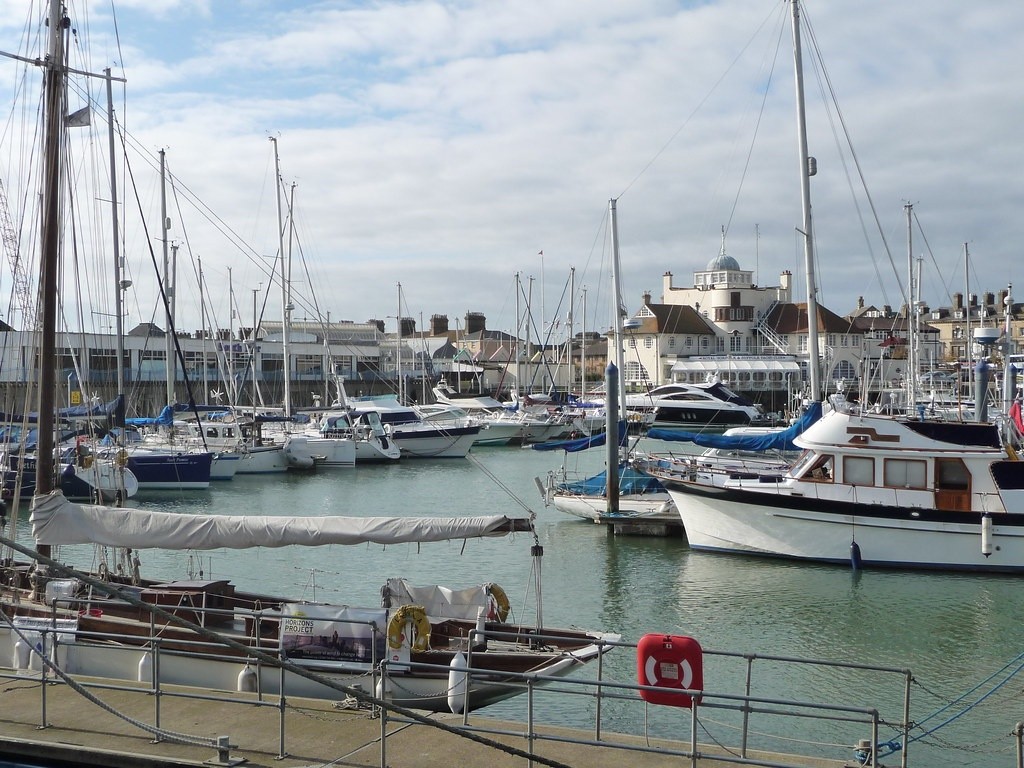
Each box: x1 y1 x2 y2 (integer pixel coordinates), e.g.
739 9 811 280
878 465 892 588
482 582 511 622
386 605 431 653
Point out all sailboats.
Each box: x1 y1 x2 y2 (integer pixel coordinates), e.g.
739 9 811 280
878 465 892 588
0 0 1024 711
